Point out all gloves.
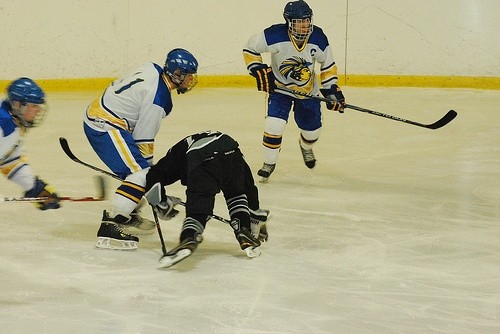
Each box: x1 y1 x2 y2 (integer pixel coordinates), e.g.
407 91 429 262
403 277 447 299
146 182 181 220
248 207 270 243
24 176 60 211
320 84 346 113
251 63 274 92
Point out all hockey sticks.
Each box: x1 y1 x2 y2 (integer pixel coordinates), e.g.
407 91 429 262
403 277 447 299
275 86 458 130
57 135 268 240
0 175 106 202
151 206 168 255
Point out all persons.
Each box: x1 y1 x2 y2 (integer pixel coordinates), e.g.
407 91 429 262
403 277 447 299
84 48 198 249
0 78 59 211
145 131 271 268
243 0 345 183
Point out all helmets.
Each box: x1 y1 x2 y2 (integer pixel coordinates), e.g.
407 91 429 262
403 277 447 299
164 48 198 95
283 0 314 41
9 77 48 128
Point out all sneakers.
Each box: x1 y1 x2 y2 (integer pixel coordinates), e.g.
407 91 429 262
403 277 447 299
230 218 262 258
157 231 203 269
121 208 157 236
258 162 275 183
95 209 140 250
299 139 316 169
